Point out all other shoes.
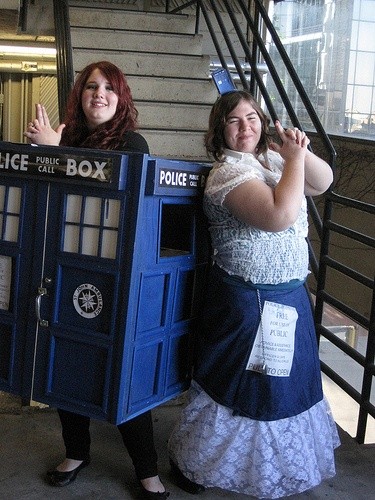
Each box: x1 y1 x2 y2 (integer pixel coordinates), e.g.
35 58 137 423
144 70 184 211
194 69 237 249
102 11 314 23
137 478 169 500
170 470 204 494
48 459 90 486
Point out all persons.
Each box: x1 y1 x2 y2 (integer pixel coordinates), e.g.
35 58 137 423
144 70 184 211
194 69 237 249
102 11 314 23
168 90 340 499
23 61 167 500
